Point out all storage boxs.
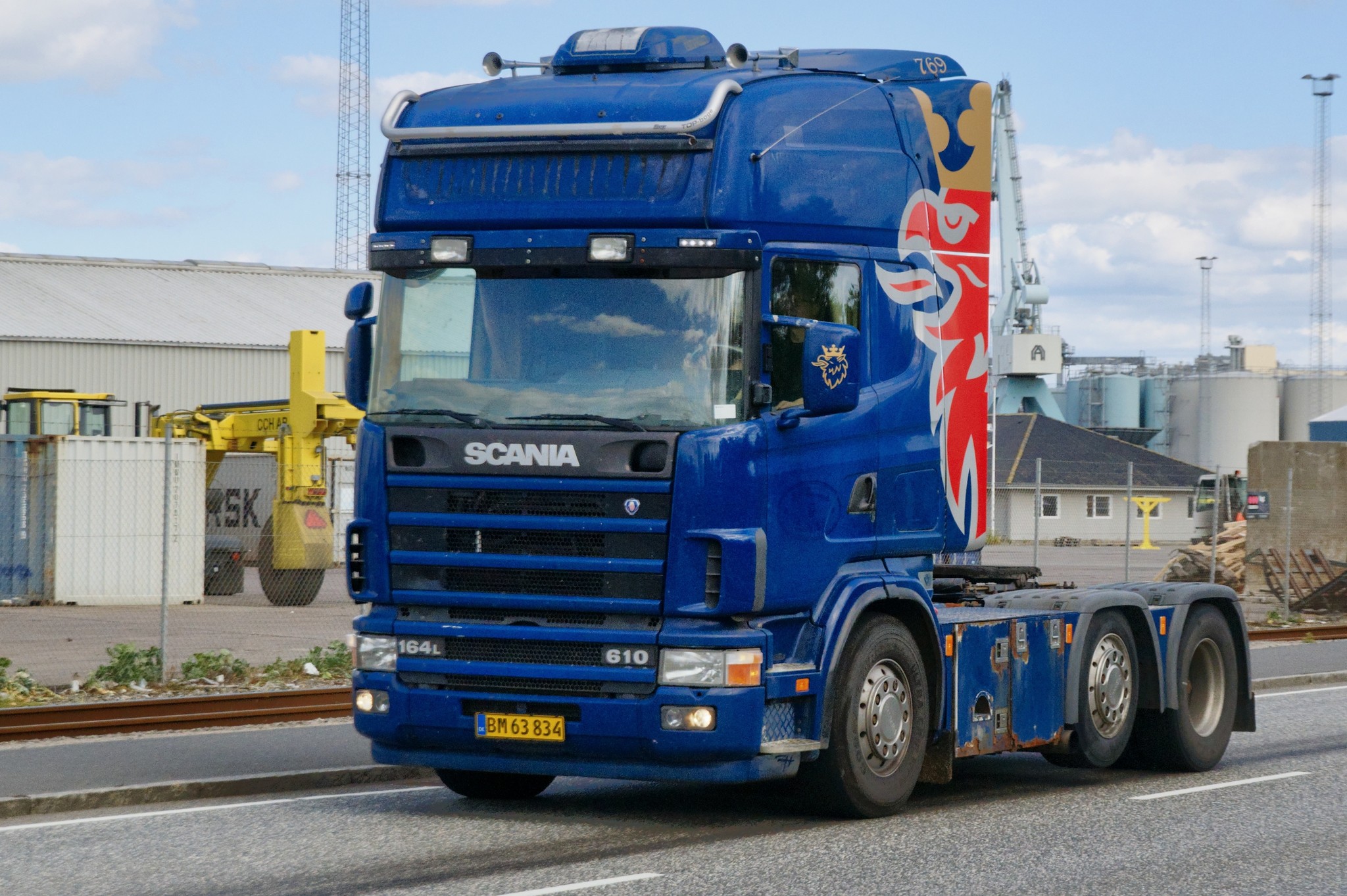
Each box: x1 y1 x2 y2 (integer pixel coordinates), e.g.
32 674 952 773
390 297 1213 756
0 435 358 606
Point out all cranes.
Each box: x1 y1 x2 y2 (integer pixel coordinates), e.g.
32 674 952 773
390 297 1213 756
988 69 1070 426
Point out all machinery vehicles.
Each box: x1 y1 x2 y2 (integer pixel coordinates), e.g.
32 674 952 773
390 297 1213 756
0 323 368 607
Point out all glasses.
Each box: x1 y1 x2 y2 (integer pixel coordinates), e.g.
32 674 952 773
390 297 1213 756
787 298 813 307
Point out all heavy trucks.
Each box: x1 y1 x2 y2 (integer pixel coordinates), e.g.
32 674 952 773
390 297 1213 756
337 22 1263 822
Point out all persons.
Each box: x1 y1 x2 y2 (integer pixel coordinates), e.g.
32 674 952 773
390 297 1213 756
772 291 820 410
